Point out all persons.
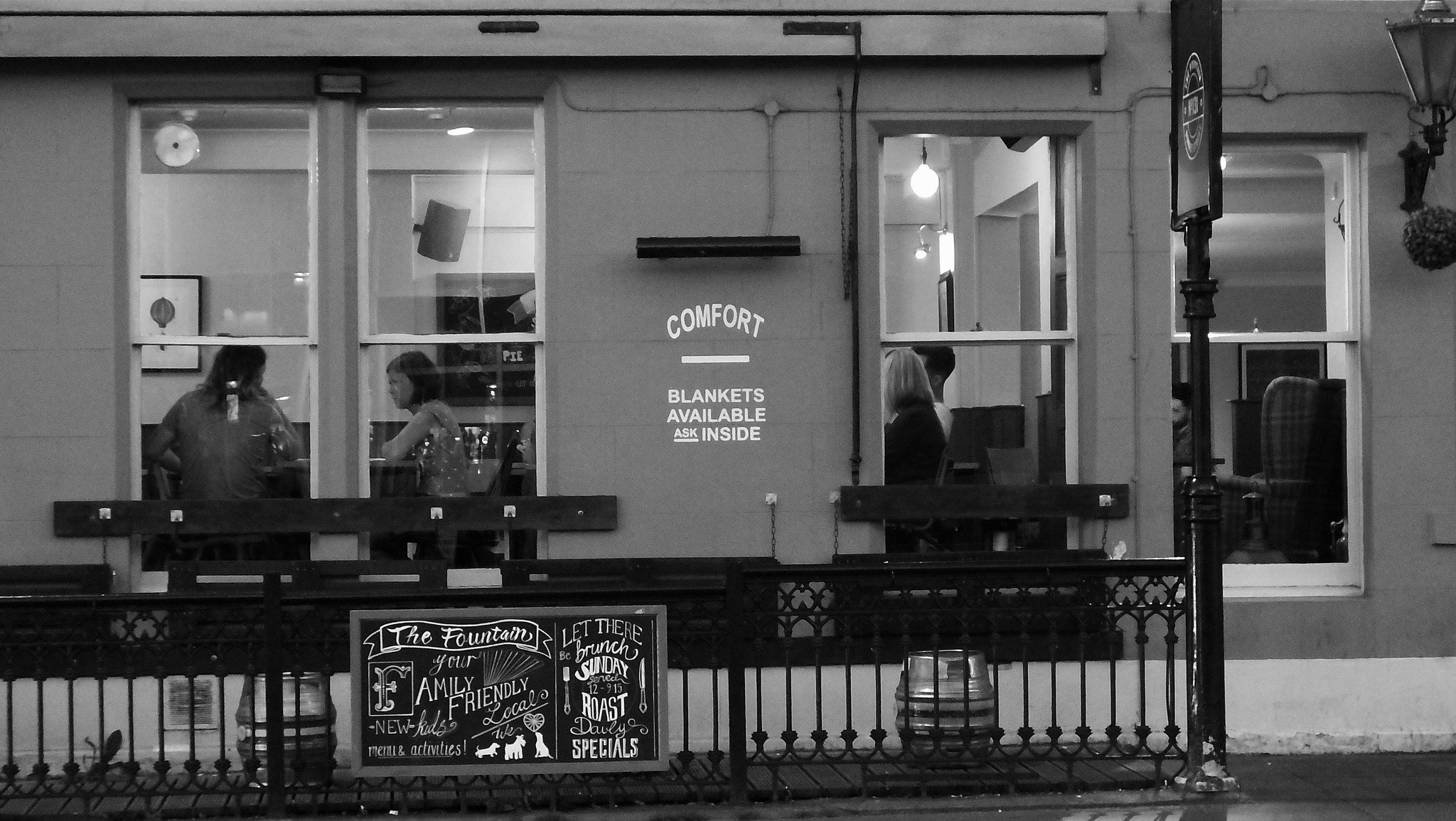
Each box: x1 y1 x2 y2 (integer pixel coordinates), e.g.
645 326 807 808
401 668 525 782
1171 383 1219 477
141 348 303 499
380 350 471 498
890 345 956 439
883 346 947 552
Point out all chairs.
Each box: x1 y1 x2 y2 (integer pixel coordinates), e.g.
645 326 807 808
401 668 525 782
152 459 268 561
405 431 519 561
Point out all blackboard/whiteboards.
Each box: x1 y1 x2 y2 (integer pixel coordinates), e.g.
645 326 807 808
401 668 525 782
347 604 673 777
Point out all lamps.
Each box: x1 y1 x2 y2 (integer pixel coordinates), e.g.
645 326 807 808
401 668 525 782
1384 0 1456 215
914 222 948 259
910 138 940 199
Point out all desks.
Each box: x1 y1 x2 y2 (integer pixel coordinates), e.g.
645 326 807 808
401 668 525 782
251 456 416 560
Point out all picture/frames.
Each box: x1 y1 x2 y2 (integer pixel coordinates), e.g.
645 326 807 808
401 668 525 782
140 275 203 374
